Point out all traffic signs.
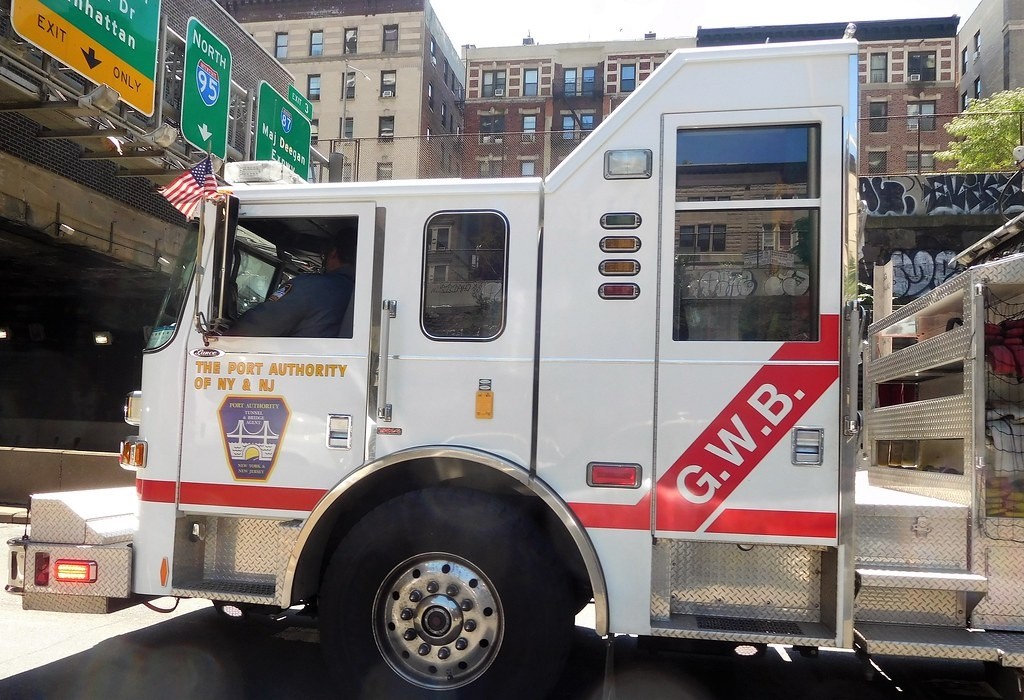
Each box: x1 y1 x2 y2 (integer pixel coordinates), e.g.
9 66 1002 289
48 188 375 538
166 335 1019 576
254 80 314 181
10 0 163 118
179 16 234 162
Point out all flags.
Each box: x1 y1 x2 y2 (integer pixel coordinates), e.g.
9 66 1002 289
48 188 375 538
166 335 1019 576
157 153 218 216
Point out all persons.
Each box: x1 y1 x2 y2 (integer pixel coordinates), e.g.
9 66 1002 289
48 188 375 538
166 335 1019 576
222 229 357 336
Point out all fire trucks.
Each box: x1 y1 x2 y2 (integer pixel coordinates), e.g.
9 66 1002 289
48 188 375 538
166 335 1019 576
0 23 1024 700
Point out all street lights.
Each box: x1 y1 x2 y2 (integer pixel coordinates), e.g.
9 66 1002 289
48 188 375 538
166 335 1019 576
340 57 372 138
